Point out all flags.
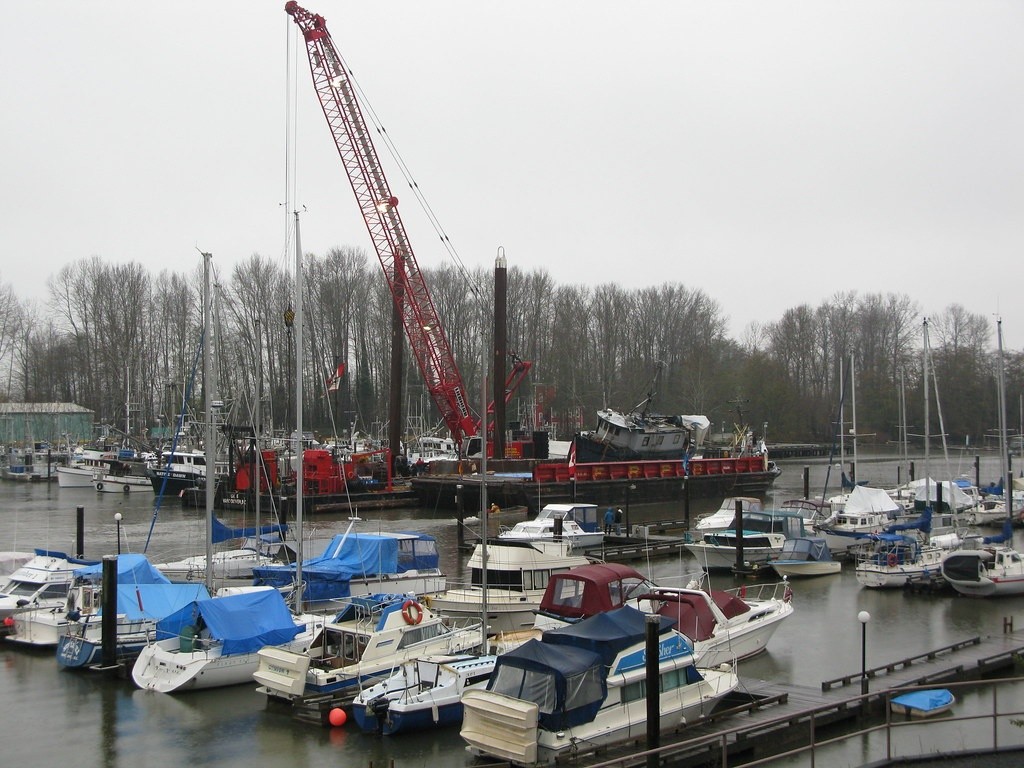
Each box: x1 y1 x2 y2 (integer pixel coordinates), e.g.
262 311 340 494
322 364 345 395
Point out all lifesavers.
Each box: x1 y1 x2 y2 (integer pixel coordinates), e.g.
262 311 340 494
124 486 130 491
888 554 898 566
402 600 423 625
97 483 103 490
348 472 354 478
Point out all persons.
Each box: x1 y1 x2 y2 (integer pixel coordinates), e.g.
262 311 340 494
603 507 614 535
416 458 424 477
615 506 624 536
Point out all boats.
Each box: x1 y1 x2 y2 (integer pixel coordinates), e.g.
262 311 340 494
888 686 956 719
458 603 739 768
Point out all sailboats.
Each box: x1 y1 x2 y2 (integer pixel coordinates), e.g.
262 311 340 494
0 203 1024 744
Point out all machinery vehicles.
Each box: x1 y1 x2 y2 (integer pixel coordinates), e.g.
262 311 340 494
286 0 537 462
